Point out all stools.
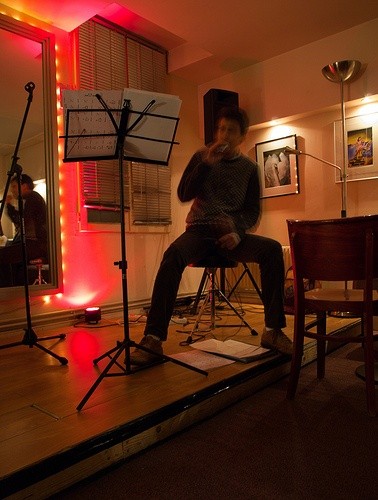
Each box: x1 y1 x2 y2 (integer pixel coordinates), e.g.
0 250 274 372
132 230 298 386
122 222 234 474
186 262 258 343
28 259 48 285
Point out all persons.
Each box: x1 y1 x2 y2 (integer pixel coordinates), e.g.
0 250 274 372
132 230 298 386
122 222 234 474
0 174 50 288
124 108 293 366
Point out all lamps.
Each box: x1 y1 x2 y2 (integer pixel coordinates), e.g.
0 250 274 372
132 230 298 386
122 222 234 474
85 307 101 324
284 59 363 318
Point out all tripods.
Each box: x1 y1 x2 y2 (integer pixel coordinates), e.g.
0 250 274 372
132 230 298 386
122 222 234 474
64 93 209 411
0 82 69 365
191 262 265 317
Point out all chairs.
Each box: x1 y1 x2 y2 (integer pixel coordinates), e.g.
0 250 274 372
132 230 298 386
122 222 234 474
286 214 378 407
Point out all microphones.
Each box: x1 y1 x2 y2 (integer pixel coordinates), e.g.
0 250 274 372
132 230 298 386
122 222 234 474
215 145 228 155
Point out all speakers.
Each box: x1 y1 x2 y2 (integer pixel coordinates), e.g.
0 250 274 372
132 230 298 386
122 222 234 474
204 88 240 146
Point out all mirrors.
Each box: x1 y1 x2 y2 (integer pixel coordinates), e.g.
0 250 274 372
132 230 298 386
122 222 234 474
0 13 63 299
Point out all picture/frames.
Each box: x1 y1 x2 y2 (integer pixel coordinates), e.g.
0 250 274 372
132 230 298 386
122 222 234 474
255 134 299 198
333 112 378 184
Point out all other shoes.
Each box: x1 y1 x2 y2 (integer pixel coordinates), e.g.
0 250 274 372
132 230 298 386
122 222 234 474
124 335 164 365
260 328 304 356
